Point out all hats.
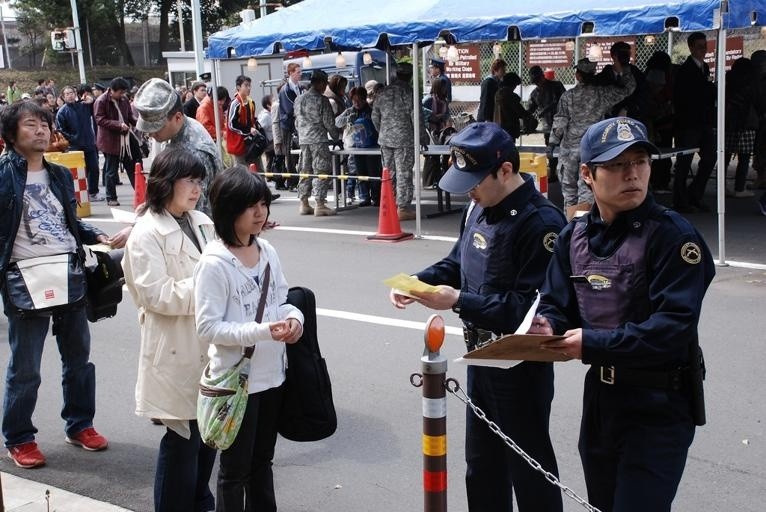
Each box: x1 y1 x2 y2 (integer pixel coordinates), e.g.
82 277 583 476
572 58 596 73
437 122 512 194
90 83 105 89
199 73 210 79
395 63 412 73
132 77 178 133
428 57 445 67
579 117 661 164
310 69 329 84
528 65 541 83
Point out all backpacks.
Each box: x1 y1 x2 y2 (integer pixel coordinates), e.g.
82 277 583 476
352 113 373 148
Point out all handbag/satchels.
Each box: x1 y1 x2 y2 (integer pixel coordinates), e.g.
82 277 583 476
245 121 269 163
196 355 250 450
85 251 124 322
4 252 88 319
138 141 148 158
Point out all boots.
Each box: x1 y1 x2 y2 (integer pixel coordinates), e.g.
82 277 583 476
314 200 335 216
299 198 313 215
396 206 415 220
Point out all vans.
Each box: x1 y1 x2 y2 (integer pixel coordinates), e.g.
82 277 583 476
284 49 398 93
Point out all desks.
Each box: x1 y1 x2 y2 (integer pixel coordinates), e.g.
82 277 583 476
289 144 464 221
516 145 702 207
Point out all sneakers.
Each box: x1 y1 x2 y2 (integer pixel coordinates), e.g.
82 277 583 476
673 181 766 215
106 200 119 206
88 193 104 202
6 441 44 468
64 426 107 451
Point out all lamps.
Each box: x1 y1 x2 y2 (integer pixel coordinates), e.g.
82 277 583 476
332 46 346 69
301 50 313 71
449 40 462 62
644 35 653 48
587 37 606 64
563 35 575 51
362 50 373 67
246 57 257 73
492 42 505 54
436 42 450 57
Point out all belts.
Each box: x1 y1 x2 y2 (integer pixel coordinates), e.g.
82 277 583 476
600 366 614 385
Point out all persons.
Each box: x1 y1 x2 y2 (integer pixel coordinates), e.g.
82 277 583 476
113 75 223 427
193 165 304 510
388 122 566 512
255 61 452 220
120 147 219 512
178 75 280 202
0 78 143 205
529 34 766 221
530 117 705 511
1 101 111 469
476 60 528 142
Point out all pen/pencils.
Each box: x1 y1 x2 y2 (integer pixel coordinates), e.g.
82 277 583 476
536 312 542 329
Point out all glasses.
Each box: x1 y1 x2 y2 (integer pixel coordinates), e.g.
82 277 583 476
591 157 651 171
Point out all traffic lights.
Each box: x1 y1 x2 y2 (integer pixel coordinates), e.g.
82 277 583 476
54 33 65 51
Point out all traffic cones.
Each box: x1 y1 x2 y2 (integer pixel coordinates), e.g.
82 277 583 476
132 162 148 210
365 166 414 242
248 163 276 231
